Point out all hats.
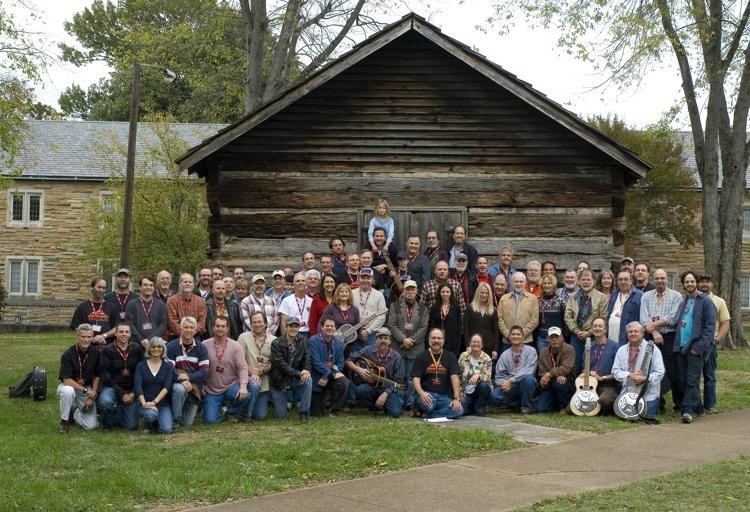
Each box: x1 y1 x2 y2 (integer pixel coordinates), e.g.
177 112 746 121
117 268 132 277
697 274 713 281
358 268 374 277
403 279 418 289
375 325 392 338
619 256 635 265
252 270 285 282
286 316 300 325
397 249 409 258
455 254 468 261
547 325 562 337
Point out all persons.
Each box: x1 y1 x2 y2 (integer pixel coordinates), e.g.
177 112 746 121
56 246 730 431
405 233 431 301
367 198 395 257
488 244 729 421
362 227 398 285
423 230 449 280
328 237 347 292
447 225 478 276
261 252 499 420
57 266 278 431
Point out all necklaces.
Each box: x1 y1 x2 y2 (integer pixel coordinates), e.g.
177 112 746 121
452 245 463 250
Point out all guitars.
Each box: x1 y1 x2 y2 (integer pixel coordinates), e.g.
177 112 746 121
569 338 601 416
380 249 408 301
337 308 388 348
352 357 405 390
614 340 654 421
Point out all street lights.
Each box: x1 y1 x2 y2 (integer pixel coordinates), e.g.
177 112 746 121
117 61 182 269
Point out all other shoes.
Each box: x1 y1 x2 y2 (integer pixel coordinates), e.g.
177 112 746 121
240 414 251 422
674 406 718 423
333 406 351 413
102 422 113 430
475 407 486 416
136 418 143 433
367 407 377 415
510 407 531 414
225 412 239 422
60 419 68 433
659 404 666 414
299 412 308 421
407 407 424 418
172 420 180 428
146 422 153 431
560 409 570 415
